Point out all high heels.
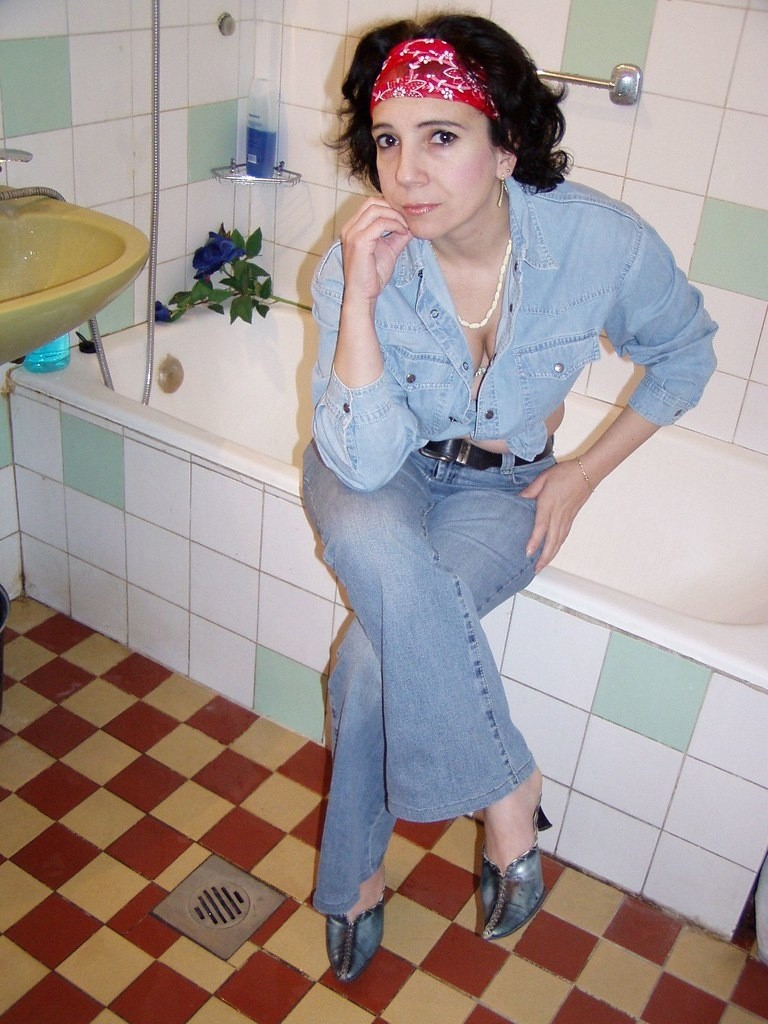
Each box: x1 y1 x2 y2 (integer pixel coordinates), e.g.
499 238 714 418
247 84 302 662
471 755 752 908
325 874 386 981
482 786 552 939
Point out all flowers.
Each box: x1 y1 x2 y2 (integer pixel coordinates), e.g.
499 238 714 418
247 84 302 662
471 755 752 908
155 222 314 323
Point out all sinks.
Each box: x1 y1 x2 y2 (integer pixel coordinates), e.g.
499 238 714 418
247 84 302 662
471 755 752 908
1 188 151 366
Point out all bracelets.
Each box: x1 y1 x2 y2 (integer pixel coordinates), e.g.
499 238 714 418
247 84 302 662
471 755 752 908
576 456 594 492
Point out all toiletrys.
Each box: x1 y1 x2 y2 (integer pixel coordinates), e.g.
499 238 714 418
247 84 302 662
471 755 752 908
24 333 71 373
245 76 279 179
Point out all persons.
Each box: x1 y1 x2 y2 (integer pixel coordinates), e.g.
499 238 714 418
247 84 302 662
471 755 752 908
302 14 718 982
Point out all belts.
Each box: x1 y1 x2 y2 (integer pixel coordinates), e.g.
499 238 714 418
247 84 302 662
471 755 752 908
419 437 554 471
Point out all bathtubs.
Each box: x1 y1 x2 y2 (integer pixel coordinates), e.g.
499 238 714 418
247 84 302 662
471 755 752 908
7 292 766 940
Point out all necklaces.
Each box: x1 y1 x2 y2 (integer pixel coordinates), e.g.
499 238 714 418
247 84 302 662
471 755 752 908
429 238 512 330
